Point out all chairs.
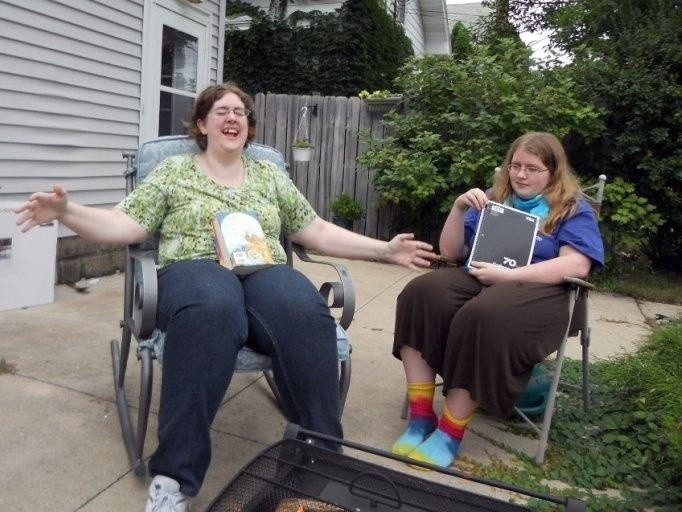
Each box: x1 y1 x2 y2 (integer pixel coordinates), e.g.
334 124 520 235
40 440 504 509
108 131 359 479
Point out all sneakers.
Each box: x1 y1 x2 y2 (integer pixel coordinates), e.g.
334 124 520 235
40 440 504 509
144 476 191 512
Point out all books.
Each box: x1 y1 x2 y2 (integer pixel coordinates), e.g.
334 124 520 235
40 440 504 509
467 200 539 272
208 210 274 272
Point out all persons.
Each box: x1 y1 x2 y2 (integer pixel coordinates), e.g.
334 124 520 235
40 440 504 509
12 80 436 512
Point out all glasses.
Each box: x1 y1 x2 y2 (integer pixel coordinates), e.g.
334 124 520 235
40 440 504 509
205 108 252 117
506 163 550 175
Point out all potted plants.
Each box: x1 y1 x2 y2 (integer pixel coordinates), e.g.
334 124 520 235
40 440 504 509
289 137 315 163
326 191 366 233
